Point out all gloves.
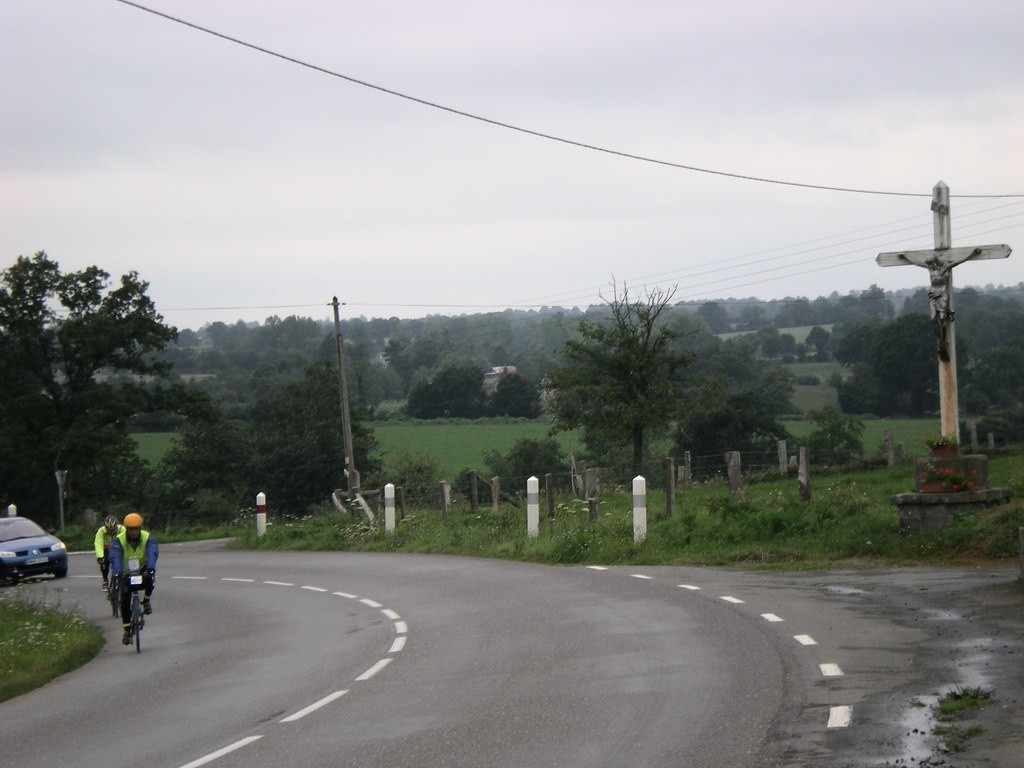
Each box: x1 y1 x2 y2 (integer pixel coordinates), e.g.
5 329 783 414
113 577 120 589
97 558 105 563
143 570 153 580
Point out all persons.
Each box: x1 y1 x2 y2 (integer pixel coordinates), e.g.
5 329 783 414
108 513 159 646
94 516 126 592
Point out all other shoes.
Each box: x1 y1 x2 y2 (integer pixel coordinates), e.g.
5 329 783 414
122 630 130 644
102 582 109 592
143 598 152 614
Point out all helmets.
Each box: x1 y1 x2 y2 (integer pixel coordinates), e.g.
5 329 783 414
104 515 117 528
124 513 143 528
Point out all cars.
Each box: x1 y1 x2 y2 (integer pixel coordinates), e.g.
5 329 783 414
0 517 69 585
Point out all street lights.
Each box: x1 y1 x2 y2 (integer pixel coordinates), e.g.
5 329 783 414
56 469 67 535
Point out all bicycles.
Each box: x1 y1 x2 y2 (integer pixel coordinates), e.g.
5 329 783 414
100 556 152 654
